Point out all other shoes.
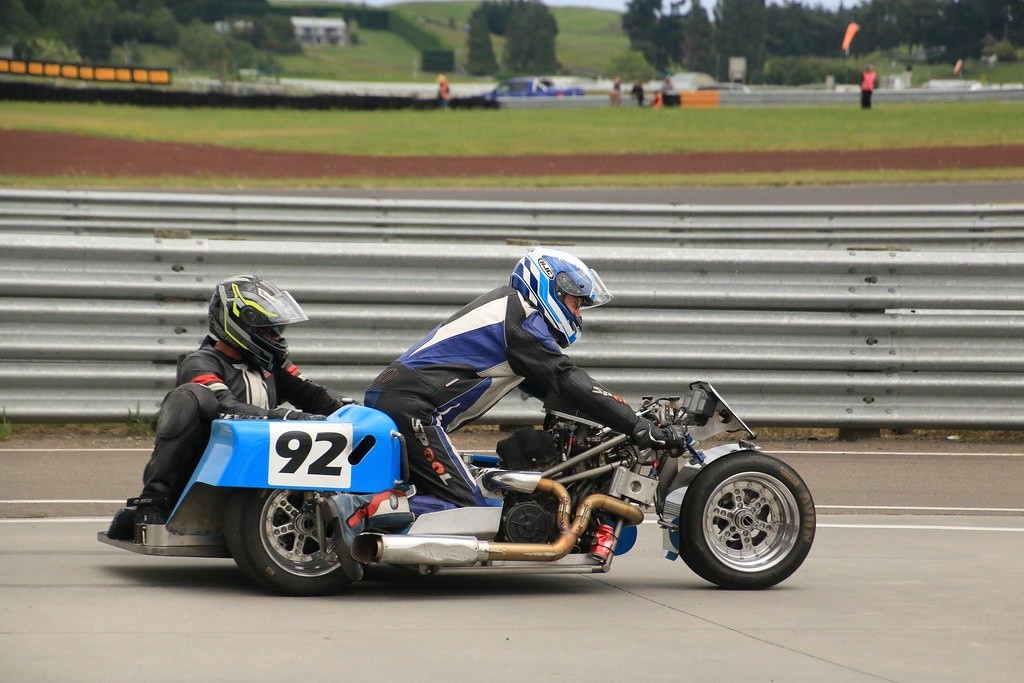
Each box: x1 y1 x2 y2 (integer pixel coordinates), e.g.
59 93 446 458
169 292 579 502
125 496 170 524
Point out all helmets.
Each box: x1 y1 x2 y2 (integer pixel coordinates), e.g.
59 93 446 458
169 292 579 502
508 248 595 349
209 275 290 373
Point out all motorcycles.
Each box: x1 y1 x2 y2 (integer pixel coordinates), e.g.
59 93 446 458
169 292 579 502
94 369 818 589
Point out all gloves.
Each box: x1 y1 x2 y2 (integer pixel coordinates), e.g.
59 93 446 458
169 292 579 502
340 398 362 406
267 408 326 421
631 417 686 458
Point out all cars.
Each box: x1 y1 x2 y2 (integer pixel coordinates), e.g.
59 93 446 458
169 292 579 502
483 78 585 98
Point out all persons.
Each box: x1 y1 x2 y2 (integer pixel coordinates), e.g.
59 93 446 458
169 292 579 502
981 52 989 85
364 248 674 519
647 77 673 108
608 77 624 106
860 63 877 108
437 74 450 110
107 274 362 539
631 80 644 106
904 64 913 82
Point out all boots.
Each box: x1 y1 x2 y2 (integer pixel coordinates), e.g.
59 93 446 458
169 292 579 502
321 485 416 581
